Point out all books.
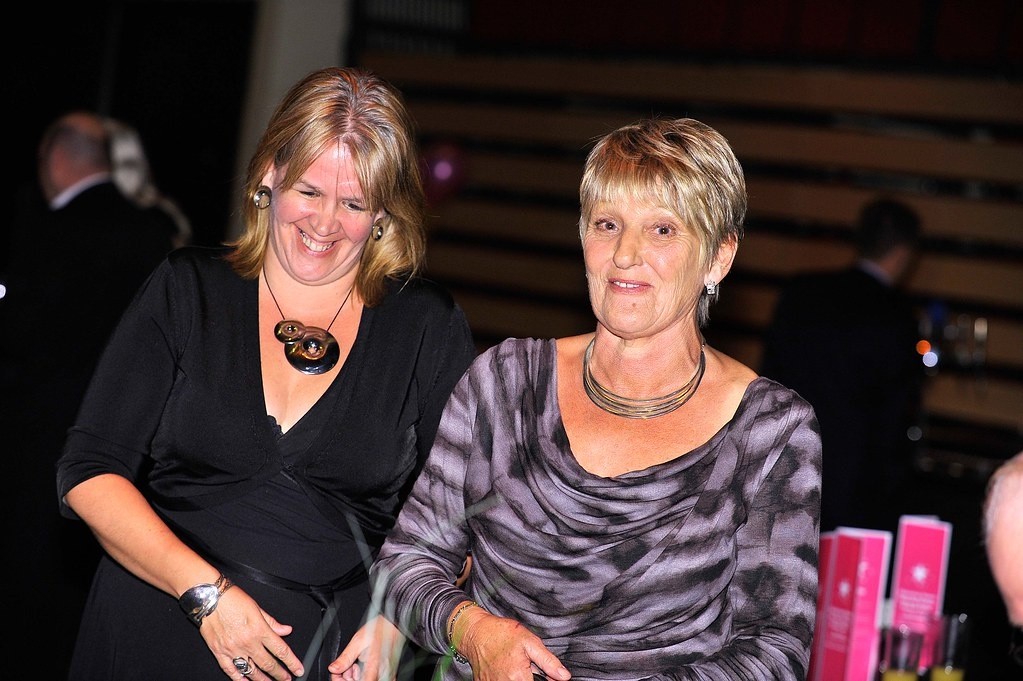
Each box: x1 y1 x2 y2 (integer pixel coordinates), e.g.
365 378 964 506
810 524 894 681
886 513 951 656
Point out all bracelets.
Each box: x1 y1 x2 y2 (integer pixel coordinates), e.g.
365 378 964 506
448 603 488 664
178 572 234 628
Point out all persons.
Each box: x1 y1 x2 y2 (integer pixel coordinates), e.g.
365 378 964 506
35 112 189 282
56 65 482 681
758 196 951 538
367 114 824 681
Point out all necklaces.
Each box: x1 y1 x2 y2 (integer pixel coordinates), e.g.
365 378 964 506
583 336 707 417
262 262 358 375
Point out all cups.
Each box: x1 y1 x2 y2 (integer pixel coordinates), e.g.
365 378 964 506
926 613 966 680
883 626 922 681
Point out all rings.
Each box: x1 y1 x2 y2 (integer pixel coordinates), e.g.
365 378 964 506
232 656 254 675
